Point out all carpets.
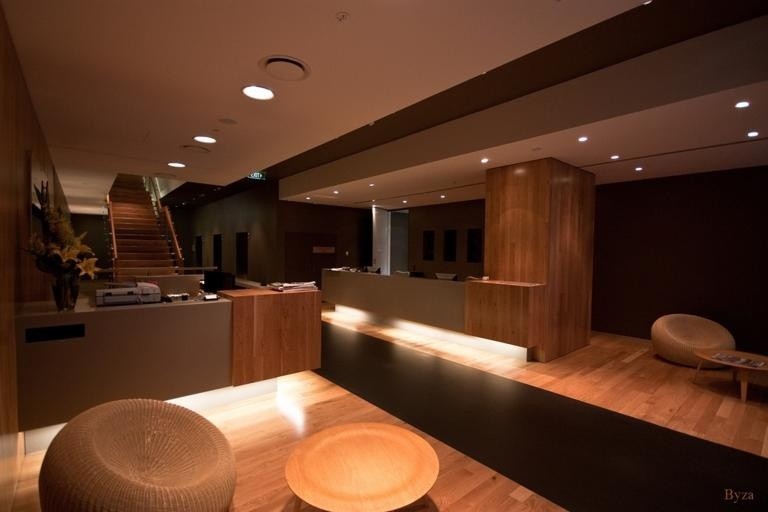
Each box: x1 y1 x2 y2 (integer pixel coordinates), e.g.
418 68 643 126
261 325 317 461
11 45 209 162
310 321 768 512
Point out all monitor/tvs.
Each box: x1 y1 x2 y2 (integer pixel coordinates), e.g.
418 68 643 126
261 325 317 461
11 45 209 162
433 272 457 281
367 266 380 273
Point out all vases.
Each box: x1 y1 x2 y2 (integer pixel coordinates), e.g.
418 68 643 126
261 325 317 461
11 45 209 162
48 274 82 309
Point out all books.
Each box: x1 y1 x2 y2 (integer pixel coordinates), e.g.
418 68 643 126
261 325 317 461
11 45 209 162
268 281 320 292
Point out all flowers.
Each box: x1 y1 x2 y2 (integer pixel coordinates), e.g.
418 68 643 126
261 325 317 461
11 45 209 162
25 178 100 307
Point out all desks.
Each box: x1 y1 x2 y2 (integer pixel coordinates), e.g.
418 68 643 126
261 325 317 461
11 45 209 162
283 421 441 512
693 348 768 403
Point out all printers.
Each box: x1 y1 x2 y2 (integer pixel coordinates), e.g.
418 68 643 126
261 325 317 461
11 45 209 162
95 282 161 306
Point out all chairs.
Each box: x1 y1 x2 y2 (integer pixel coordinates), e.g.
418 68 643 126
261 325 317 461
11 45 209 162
652 313 737 370
37 396 237 512
